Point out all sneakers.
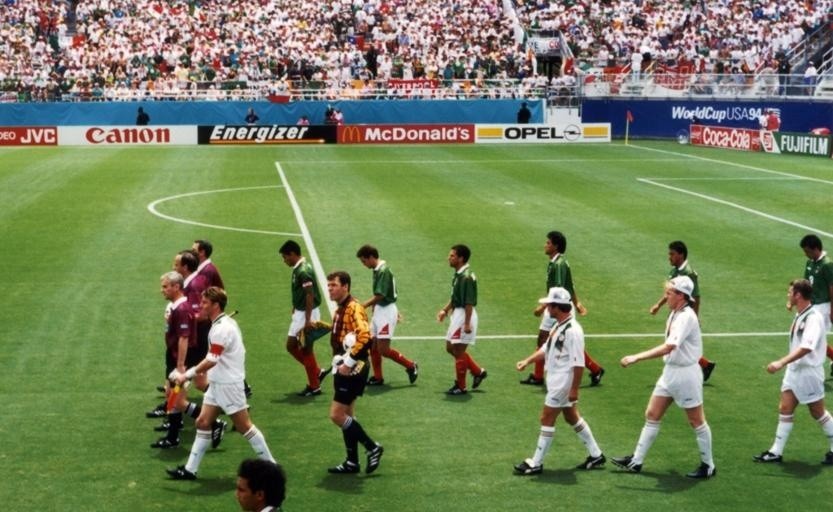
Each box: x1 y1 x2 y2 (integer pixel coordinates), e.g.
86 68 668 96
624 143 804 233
318 367 328 384
405 362 419 385
518 372 545 388
512 456 544 476
575 452 607 471
164 465 198 482
296 385 322 399
471 367 489 392
244 378 252 399
143 383 185 448
701 360 716 383
588 366 605 387
365 443 384 475
443 380 468 396
211 419 228 451
327 460 361 475
686 460 717 480
364 376 385 387
753 449 784 464
609 452 643 474
820 450 833 467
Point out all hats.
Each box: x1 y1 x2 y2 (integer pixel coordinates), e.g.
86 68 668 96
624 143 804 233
536 285 575 305
665 274 697 304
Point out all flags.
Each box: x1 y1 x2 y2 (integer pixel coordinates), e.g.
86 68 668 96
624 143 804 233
627 111 633 122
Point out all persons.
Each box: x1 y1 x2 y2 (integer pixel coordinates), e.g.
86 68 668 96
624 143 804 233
518 102 531 124
759 111 770 129
330 108 343 126
137 107 149 125
296 115 311 126
691 113 699 124
245 109 258 125
808 128 830 135
766 111 781 131
324 104 333 125
